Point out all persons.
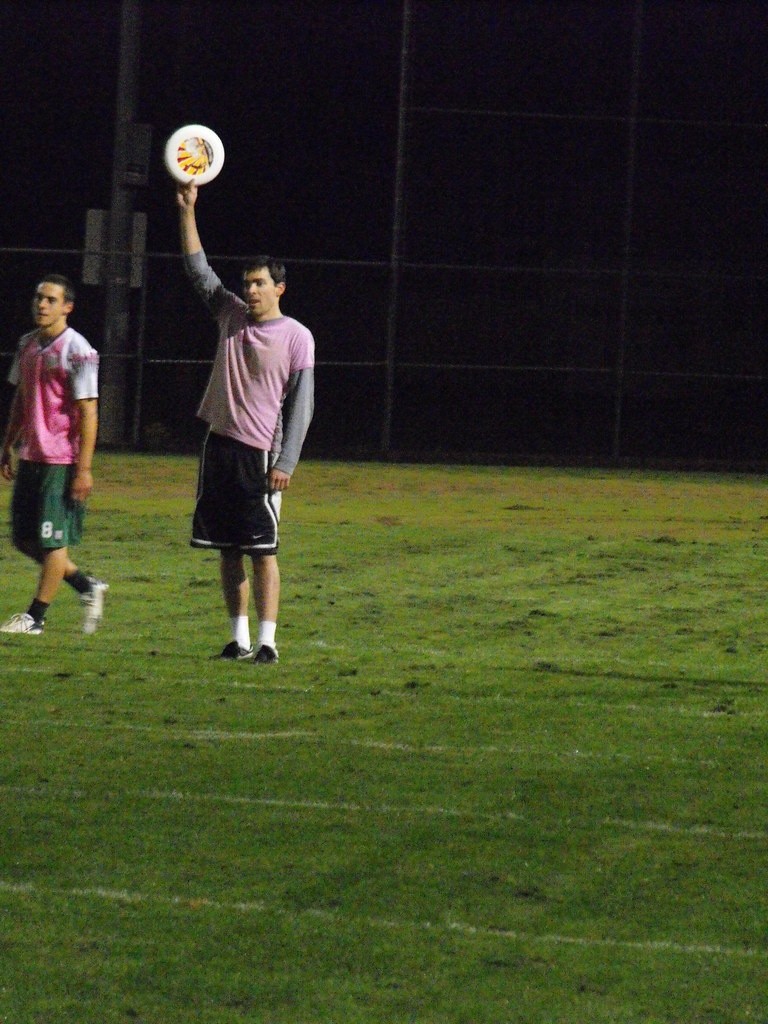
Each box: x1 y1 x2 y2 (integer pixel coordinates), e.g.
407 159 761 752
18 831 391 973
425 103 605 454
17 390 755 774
177 178 314 662
0 272 110 636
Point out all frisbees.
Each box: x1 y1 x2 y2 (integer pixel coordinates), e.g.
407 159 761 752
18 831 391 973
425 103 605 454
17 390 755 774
164 123 225 186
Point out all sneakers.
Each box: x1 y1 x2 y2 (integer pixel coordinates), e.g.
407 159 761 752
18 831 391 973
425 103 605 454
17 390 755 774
79 575 109 634
0 612 47 635
210 641 254 663
253 645 280 666
